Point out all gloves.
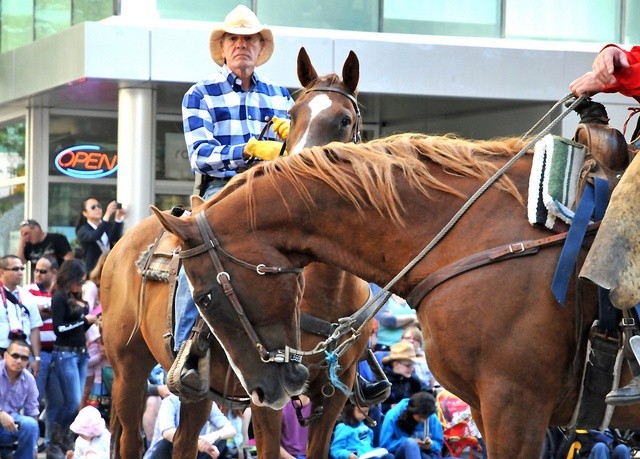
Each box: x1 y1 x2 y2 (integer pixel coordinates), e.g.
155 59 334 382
272 116 291 140
244 136 288 161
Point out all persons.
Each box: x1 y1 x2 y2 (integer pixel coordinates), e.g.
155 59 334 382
241 407 256 455
25 254 72 453
65 406 112 459
374 292 421 352
170 4 398 407
83 279 170 458
378 391 444 459
0 339 41 459
567 41 639 407
586 429 631 459
76 197 127 276
143 393 236 459
46 257 103 459
380 341 433 415
0 255 41 377
15 218 76 262
280 394 313 458
400 327 435 391
329 403 396 459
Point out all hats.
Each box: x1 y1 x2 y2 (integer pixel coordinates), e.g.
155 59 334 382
70 405 105 436
382 342 425 363
209 5 274 67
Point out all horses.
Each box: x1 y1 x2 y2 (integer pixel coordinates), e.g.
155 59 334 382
146 132 640 459
98 46 373 459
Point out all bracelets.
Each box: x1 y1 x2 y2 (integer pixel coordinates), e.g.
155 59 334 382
34 357 40 360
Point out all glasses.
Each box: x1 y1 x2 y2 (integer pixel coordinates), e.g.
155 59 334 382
35 269 48 274
7 351 29 361
91 204 103 209
4 267 24 271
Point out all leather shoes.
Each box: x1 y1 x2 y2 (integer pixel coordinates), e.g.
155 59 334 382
358 373 392 400
180 356 200 391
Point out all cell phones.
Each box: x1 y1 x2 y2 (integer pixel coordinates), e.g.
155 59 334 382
96 312 102 318
424 442 430 445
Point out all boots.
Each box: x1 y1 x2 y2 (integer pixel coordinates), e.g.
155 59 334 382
605 309 639 405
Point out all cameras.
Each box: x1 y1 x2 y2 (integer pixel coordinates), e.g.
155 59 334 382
8 329 27 342
116 203 121 209
